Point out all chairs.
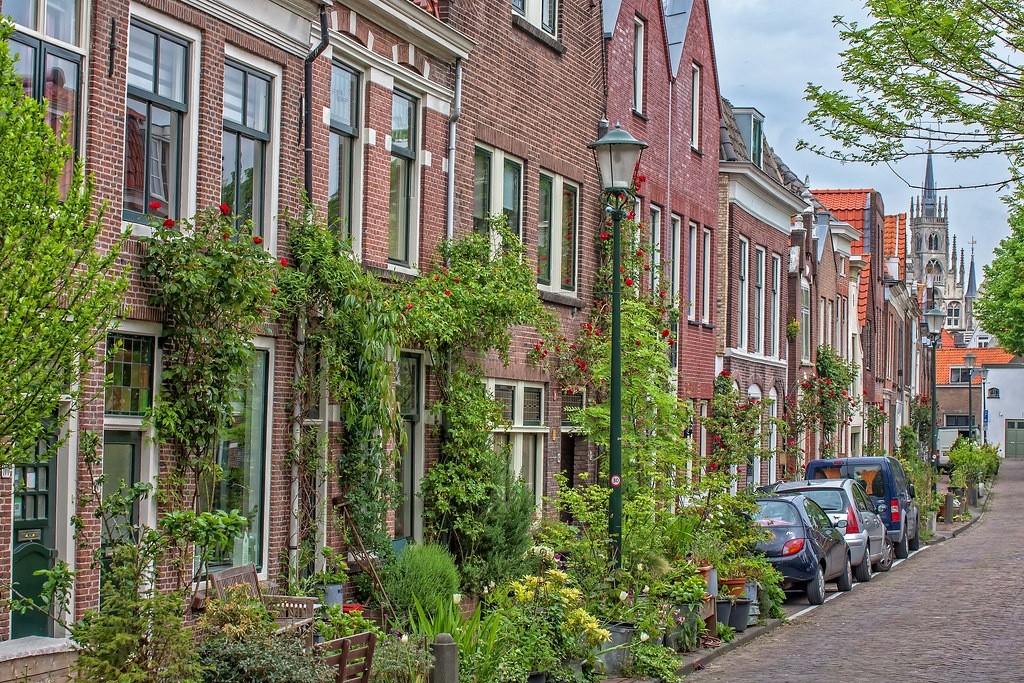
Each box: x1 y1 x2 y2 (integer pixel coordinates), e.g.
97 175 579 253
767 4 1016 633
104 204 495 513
209 562 319 649
313 633 377 683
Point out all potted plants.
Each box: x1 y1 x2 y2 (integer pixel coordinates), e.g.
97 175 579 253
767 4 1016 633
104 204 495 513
315 546 350 616
937 492 945 516
528 465 783 683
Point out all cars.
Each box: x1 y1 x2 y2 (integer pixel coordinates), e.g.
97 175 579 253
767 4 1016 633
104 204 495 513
771 478 894 582
736 494 854 604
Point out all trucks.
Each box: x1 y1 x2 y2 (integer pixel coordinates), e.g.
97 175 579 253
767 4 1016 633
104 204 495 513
936 429 960 473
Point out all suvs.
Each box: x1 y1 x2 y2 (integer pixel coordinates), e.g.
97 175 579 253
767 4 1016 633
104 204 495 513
803 455 922 560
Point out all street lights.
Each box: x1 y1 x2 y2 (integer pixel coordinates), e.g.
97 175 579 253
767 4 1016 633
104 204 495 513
961 351 977 453
978 363 991 448
921 305 946 497
583 121 652 602
